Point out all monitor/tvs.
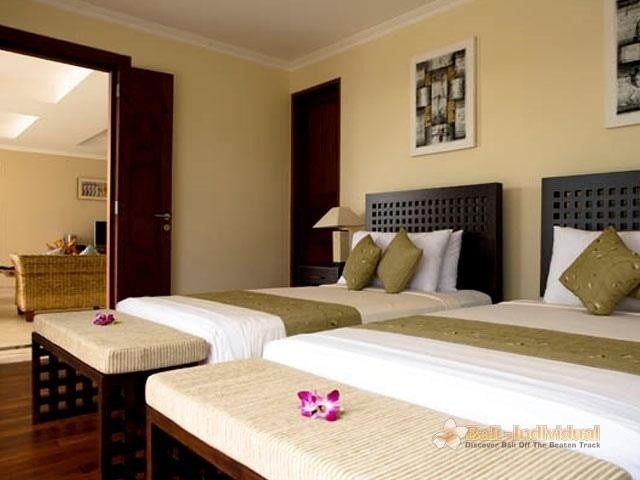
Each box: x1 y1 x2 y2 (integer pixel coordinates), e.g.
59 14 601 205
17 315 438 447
93 219 107 248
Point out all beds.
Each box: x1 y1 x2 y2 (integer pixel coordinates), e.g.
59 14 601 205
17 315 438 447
115 183 504 478
261 172 640 478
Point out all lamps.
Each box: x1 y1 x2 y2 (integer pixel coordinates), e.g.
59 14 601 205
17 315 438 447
314 207 365 264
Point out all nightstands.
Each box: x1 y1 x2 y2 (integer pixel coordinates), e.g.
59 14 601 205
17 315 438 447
295 267 344 286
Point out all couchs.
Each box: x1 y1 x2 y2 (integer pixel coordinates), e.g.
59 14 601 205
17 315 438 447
10 255 107 321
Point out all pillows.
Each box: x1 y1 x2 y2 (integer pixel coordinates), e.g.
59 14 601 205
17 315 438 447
437 228 465 294
41 248 64 255
558 224 640 316
379 227 423 295
337 233 379 292
78 247 98 256
542 225 640 311
354 228 454 293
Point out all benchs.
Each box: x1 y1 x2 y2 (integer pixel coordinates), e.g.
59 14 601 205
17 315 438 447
31 311 209 479
144 359 633 478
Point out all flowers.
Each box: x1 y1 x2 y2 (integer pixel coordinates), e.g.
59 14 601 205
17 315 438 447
91 312 118 325
296 387 346 422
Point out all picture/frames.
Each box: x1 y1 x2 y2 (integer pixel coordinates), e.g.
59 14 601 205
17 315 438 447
411 36 478 157
77 177 108 201
602 1 640 129
